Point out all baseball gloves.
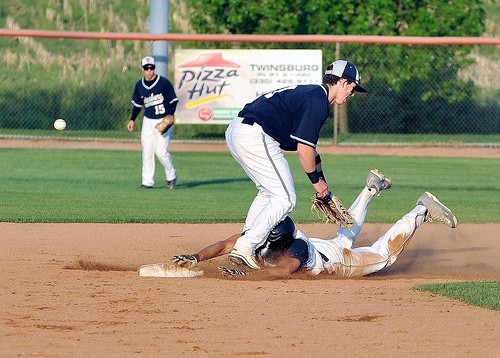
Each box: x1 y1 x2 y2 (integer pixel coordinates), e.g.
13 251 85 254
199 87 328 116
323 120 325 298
154 115 174 135
312 192 353 229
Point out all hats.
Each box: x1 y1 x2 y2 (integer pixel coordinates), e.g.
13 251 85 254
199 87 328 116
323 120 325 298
141 56 155 66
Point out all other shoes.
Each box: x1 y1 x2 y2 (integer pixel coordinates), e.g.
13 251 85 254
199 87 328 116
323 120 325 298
227 246 277 272
166 178 176 188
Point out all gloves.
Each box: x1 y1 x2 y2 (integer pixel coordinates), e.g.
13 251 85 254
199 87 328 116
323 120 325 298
217 265 250 277
172 254 198 270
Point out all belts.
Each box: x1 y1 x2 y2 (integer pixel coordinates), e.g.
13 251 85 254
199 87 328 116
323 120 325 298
242 119 254 126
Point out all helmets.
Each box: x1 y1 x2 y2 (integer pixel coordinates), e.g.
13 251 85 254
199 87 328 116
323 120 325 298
325 59 368 94
267 215 297 251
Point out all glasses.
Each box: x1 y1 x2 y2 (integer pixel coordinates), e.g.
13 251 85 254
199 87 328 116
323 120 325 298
143 66 155 71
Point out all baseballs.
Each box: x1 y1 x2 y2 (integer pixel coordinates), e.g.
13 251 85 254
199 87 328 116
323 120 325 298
54 119 67 130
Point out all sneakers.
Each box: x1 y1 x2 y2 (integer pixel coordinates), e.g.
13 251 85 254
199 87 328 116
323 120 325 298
416 191 458 228
365 169 392 198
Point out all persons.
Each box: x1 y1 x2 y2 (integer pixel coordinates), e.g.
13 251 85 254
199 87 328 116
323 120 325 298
127 56 179 190
225 58 369 272
169 169 459 280
